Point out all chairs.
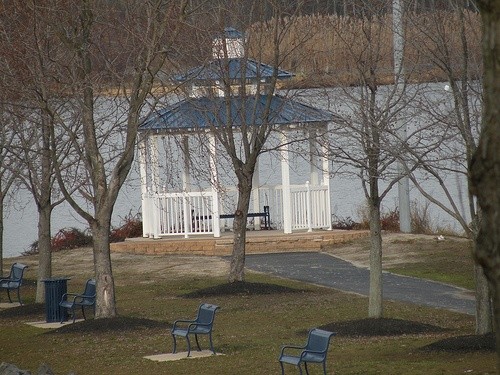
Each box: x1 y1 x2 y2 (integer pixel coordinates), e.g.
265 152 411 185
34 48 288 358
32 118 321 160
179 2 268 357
278 328 337 375
58 278 98 323
0 263 29 305
171 301 220 357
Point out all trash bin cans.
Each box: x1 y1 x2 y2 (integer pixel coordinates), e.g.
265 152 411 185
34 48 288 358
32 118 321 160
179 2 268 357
40 279 71 322
